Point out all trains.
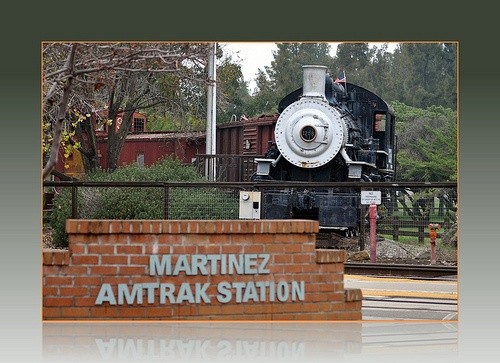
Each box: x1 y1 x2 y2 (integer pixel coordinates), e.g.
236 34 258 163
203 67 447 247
54 61 399 235
